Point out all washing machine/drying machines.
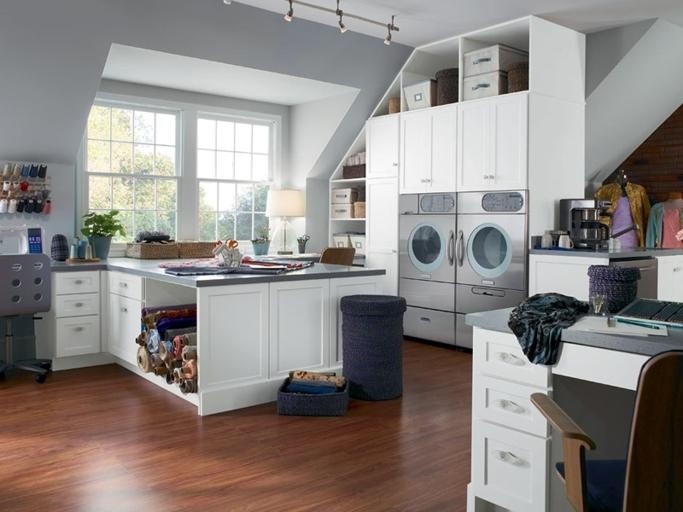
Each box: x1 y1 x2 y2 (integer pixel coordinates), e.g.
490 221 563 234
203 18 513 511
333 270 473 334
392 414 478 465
456 190 528 315
397 193 457 314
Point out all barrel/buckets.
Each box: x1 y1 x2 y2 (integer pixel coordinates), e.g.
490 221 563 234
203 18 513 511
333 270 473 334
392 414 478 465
434 68 458 106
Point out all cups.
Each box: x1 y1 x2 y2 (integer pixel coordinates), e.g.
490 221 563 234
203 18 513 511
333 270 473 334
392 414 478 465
558 234 574 249
298 243 305 253
541 234 556 249
388 98 399 113
352 201 364 218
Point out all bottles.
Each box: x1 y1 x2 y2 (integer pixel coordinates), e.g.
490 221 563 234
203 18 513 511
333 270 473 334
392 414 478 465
68 240 93 260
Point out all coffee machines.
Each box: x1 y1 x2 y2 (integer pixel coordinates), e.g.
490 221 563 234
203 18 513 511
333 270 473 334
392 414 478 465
558 199 611 251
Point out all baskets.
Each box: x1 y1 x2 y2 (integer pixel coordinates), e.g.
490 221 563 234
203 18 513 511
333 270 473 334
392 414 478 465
178 242 223 258
125 242 178 258
277 376 352 417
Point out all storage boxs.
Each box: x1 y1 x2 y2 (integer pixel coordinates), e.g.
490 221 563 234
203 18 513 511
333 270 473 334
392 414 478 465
464 44 529 99
332 164 365 257
403 79 436 109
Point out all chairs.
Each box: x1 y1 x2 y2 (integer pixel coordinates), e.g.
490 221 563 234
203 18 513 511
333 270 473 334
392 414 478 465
530 351 683 512
320 248 355 265
0 254 51 383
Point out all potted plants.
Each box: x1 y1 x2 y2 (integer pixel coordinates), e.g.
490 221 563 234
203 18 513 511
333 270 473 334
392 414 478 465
249 224 271 254
81 206 124 258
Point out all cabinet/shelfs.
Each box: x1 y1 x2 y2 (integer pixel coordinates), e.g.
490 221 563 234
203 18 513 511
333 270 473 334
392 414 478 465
528 250 659 303
329 177 365 267
102 268 202 416
404 105 457 194
461 96 530 191
365 116 399 296
36 265 102 372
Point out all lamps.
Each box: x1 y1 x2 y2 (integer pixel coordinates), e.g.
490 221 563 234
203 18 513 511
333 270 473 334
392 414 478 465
283 0 400 47
266 190 307 254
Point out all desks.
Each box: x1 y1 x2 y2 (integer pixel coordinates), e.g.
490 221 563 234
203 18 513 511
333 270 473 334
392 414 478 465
465 300 683 512
